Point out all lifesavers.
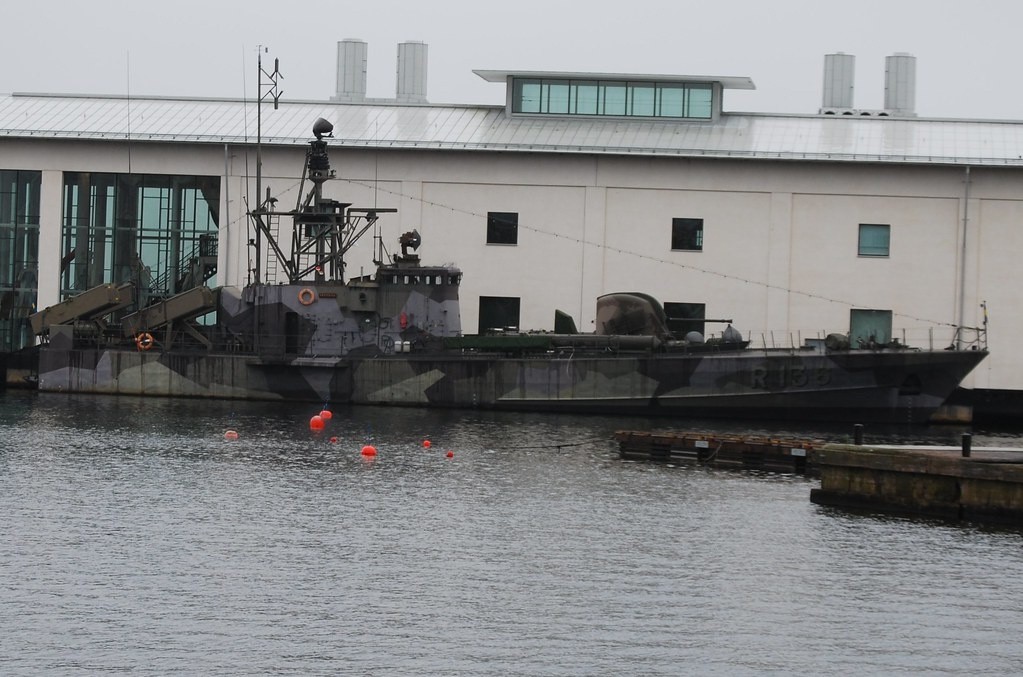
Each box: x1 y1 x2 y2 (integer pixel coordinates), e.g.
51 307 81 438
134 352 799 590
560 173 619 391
299 288 315 305
137 333 153 351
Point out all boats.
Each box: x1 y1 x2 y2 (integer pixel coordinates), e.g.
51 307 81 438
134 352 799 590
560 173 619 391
22 42 989 420
666 324 752 350
